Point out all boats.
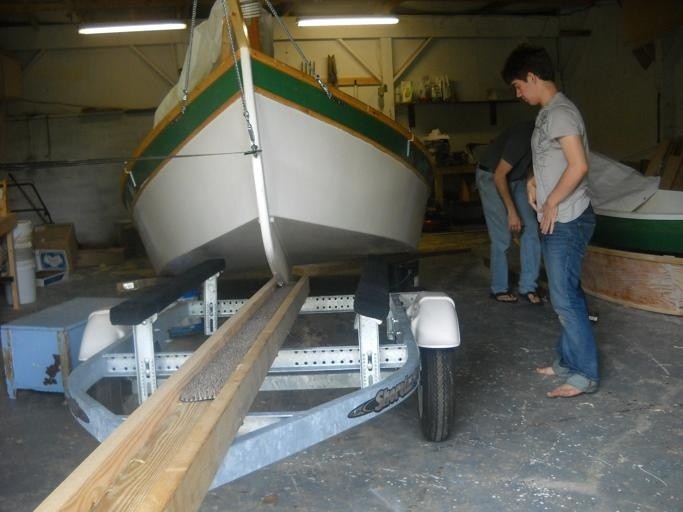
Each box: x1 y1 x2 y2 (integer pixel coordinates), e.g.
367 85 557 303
118 1 437 278
585 187 683 255
511 235 682 316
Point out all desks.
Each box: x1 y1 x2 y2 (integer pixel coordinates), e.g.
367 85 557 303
430 163 478 210
0 211 20 311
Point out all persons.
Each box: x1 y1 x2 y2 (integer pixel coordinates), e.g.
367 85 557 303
475 117 544 304
500 40 601 399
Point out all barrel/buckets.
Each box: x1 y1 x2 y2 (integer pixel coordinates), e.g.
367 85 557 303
422 134 449 165
13 220 33 249
3 260 36 305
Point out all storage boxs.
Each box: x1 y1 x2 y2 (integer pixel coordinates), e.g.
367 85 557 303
0 295 132 404
29 221 78 275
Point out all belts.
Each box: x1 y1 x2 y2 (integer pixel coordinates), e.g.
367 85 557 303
476 162 524 182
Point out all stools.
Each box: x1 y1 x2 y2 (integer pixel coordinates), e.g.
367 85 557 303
112 214 143 259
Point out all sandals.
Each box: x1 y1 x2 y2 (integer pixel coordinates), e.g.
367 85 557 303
516 289 545 306
488 291 518 303
